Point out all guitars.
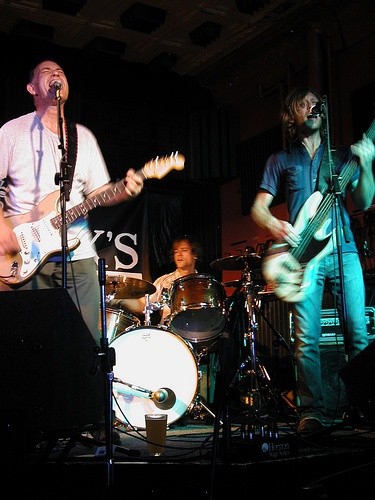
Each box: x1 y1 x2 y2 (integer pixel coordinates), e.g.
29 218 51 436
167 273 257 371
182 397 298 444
261 120 375 303
0 151 186 286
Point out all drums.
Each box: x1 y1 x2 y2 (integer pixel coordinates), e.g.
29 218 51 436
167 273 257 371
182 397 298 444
105 325 198 430
169 274 228 344
99 306 141 346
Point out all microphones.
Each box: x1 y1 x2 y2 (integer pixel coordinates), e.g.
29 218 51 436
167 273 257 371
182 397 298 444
50 80 61 89
152 391 165 402
310 95 326 116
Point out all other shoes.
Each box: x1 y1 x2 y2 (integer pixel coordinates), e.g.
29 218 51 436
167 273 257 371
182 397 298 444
92 428 120 444
297 417 325 435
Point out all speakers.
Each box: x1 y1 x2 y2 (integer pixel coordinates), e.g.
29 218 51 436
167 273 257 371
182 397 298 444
295 339 375 432
0 287 110 438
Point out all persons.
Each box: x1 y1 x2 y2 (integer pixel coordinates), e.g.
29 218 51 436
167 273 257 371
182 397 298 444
0 59 144 446
248 89 375 432
111 236 217 420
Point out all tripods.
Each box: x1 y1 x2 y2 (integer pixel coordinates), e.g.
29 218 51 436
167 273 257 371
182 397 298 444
218 261 281 413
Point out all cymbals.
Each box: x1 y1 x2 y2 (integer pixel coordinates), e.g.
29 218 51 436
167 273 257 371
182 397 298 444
102 275 156 299
209 254 248 272
